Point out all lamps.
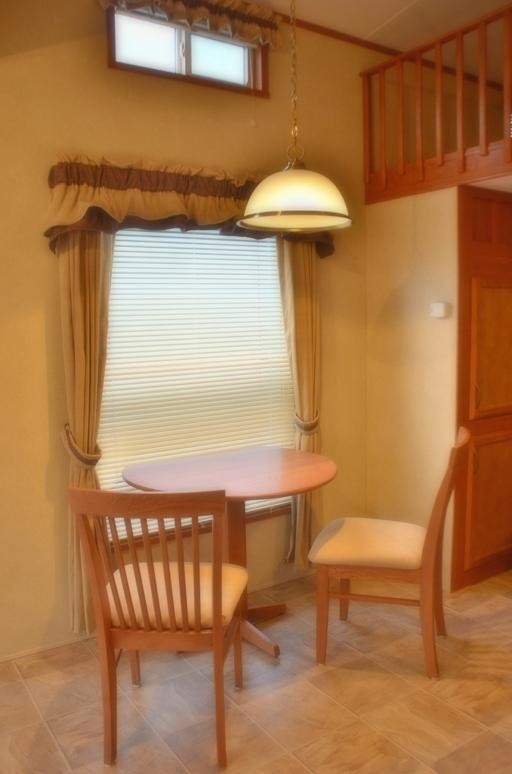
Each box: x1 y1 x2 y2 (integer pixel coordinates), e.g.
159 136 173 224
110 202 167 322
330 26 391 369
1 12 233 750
234 0 352 232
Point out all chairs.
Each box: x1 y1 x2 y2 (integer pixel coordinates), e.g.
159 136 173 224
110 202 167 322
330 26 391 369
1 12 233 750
307 426 473 679
66 485 249 770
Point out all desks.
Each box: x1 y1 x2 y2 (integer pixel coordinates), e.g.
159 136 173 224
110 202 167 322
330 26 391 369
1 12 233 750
124 447 339 657
